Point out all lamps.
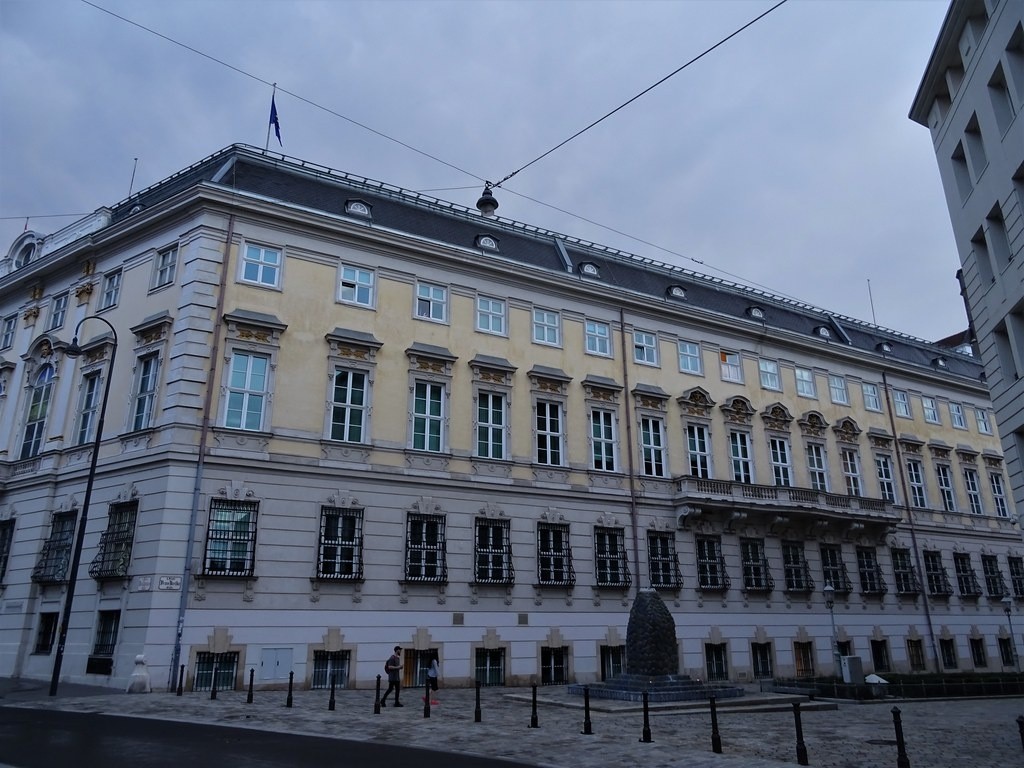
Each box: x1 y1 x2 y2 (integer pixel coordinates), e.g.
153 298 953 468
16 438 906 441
476 182 499 217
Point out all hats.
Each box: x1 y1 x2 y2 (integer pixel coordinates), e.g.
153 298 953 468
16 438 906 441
394 646 403 651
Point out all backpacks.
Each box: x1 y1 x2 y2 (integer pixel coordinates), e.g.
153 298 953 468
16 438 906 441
385 655 397 674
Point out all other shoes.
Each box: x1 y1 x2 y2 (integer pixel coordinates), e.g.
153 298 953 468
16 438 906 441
394 703 403 707
431 700 440 704
380 699 386 707
422 695 426 702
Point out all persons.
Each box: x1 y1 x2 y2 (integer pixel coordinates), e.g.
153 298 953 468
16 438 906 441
422 650 440 704
380 646 404 707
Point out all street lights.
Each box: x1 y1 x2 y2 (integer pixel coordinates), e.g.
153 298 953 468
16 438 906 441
1001 593 1021 674
823 579 837 685
39 314 119 705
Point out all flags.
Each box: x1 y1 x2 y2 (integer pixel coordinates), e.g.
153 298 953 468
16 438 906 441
270 96 283 146
